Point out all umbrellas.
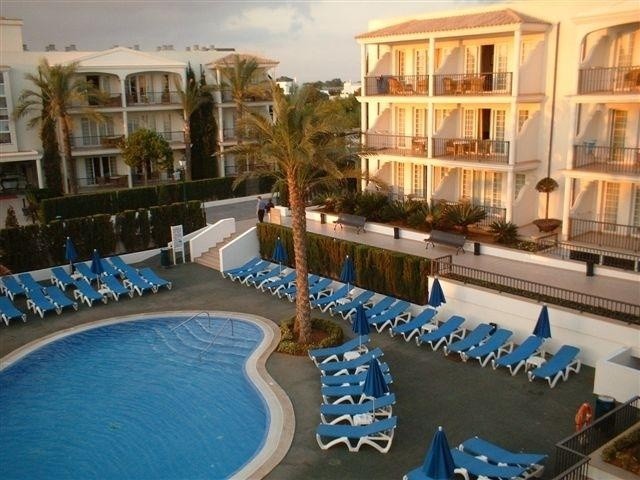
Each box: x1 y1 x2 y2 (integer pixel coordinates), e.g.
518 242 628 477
272 236 288 274
362 352 388 421
65 236 77 275
89 249 104 289
429 275 446 316
352 301 371 350
340 255 355 293
532 303 552 358
422 426 456 479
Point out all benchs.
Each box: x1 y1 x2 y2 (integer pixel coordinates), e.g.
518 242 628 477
332 212 367 235
424 229 467 255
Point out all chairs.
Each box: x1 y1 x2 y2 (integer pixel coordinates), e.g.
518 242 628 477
328 289 375 320
0 255 174 327
247 264 287 293
261 269 300 297
441 75 486 95
385 77 428 96
461 327 514 368
221 255 264 282
365 299 412 336
442 322 494 357
388 307 440 344
402 436 549 480
443 138 492 162
526 344 582 388
491 335 546 377
309 283 354 314
307 331 398 453
286 277 334 300
278 275 320 300
348 296 397 328
414 314 468 353
232 261 272 287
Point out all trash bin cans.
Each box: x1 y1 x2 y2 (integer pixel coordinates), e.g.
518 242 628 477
321 214 327 224
587 260 593 276
474 243 480 255
161 250 170 269
394 227 399 239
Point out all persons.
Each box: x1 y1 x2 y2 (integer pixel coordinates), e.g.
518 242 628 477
256 197 266 223
264 199 274 222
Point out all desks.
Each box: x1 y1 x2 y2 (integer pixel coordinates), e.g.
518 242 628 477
335 297 350 307
420 323 438 336
525 355 546 374
267 276 282 284
